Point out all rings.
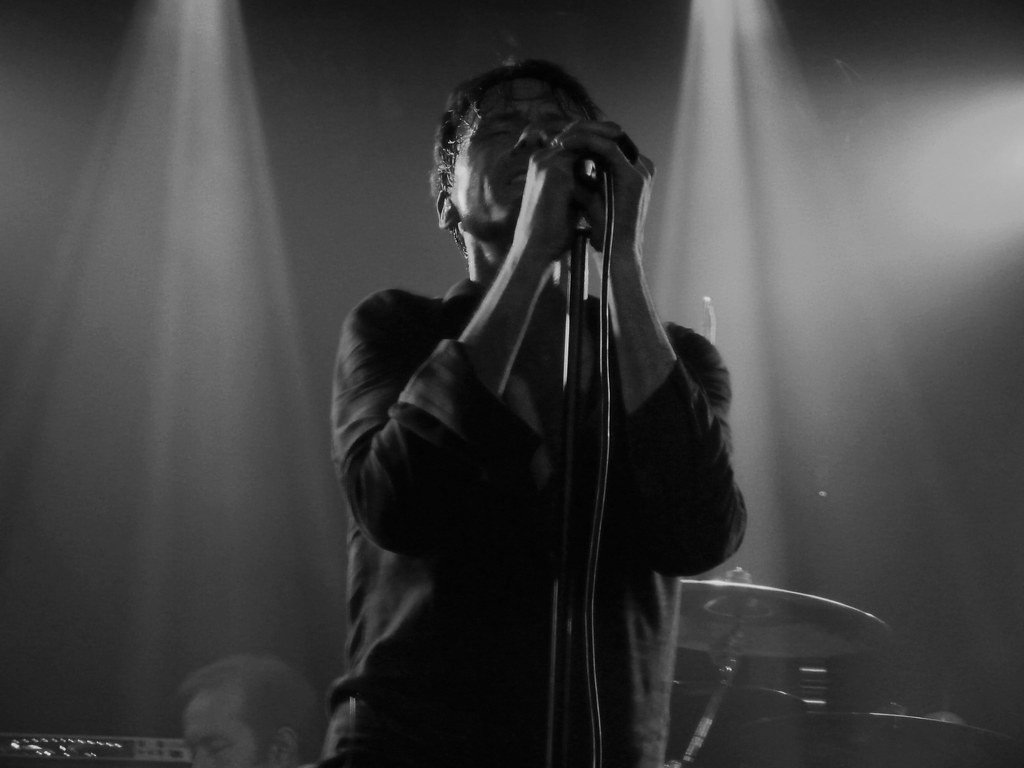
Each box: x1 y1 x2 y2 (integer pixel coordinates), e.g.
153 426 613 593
612 130 641 166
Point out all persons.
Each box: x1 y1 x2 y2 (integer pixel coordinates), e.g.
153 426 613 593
313 59 750 768
182 655 305 768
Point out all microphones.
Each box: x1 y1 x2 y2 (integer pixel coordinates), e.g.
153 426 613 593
574 149 613 179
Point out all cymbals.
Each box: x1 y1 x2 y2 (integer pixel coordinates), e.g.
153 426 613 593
677 579 891 658
675 685 811 713
